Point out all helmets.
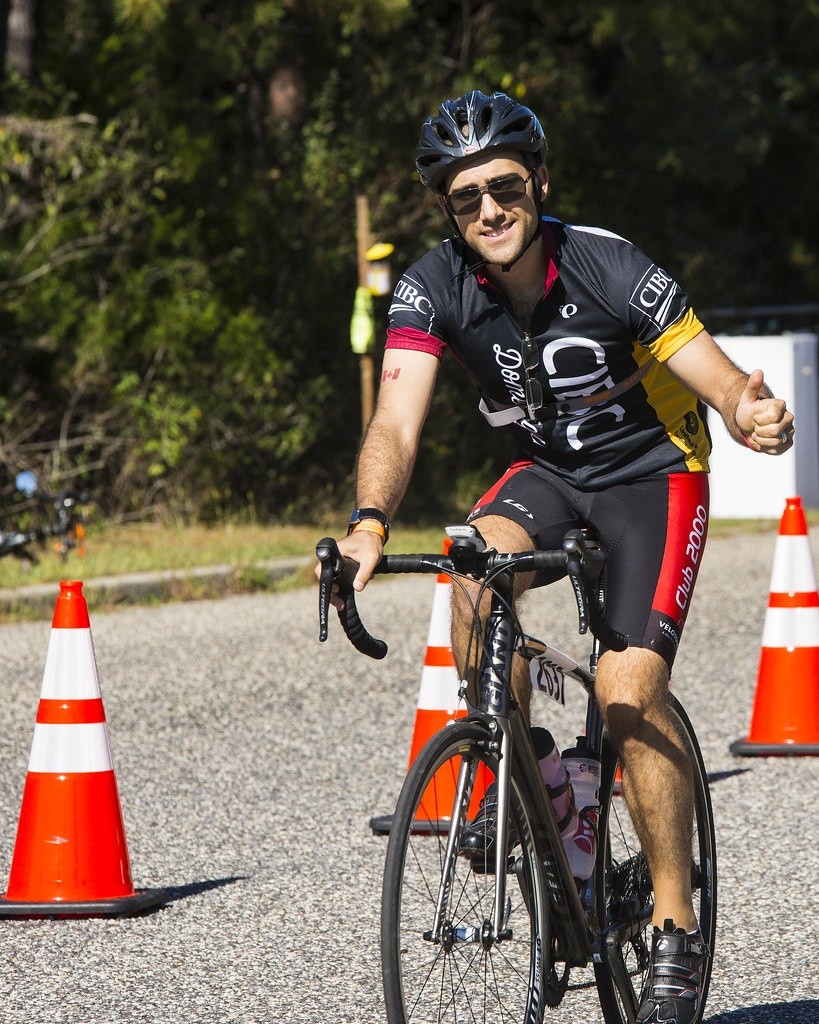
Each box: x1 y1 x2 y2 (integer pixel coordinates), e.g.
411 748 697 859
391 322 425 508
416 89 548 199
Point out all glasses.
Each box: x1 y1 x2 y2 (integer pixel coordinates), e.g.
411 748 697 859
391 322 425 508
521 331 543 415
442 171 534 216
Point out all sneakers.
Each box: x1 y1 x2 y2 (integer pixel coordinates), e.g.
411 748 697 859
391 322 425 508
460 780 520 861
633 918 707 1024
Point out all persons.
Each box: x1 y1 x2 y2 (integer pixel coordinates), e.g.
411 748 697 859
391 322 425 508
315 90 798 1022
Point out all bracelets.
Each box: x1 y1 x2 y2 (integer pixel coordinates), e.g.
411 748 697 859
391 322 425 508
740 430 754 450
346 507 390 546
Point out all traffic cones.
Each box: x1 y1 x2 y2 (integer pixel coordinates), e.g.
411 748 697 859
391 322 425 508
0 580 166 916
369 538 493 834
729 497 819 757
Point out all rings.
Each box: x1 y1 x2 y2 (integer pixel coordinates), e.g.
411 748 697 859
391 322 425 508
780 433 787 445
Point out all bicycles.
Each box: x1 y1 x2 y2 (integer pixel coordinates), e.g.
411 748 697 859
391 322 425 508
316 524 718 1024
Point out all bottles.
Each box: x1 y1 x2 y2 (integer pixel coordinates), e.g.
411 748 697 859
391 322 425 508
561 736 601 881
528 727 579 837
